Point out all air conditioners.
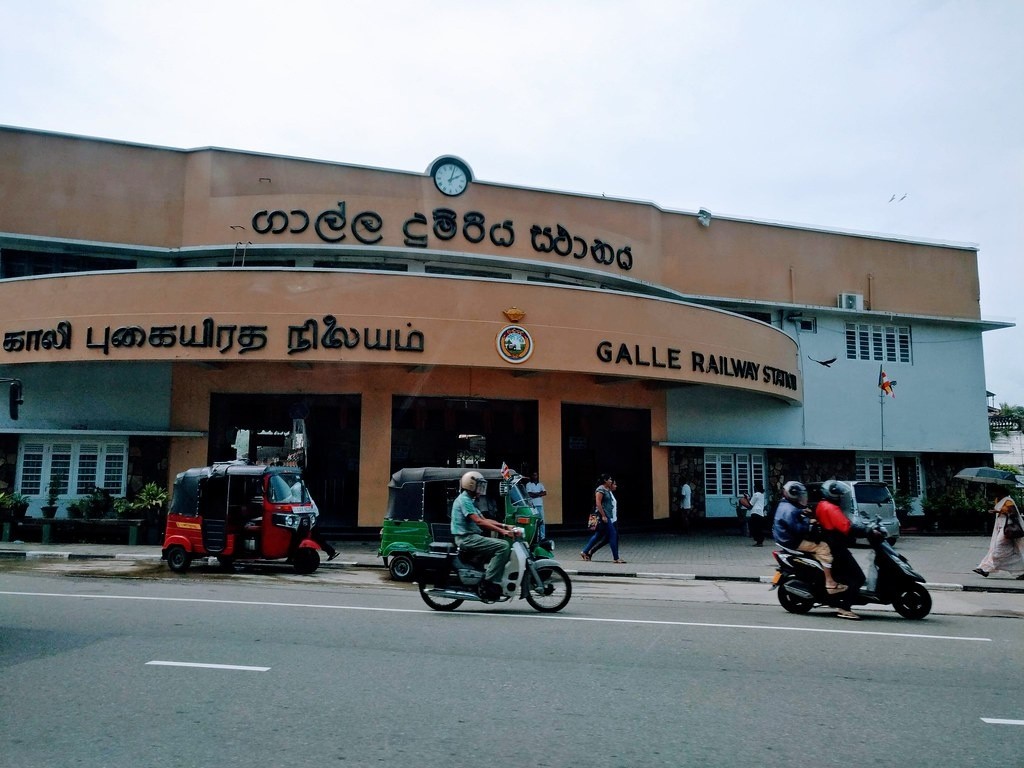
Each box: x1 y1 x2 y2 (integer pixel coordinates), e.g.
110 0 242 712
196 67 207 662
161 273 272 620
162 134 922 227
837 293 864 309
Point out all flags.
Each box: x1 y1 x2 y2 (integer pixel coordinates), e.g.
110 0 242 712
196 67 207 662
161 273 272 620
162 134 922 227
500 462 512 482
878 369 895 399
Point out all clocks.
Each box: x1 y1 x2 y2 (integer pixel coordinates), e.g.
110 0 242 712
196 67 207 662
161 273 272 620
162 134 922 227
433 162 469 196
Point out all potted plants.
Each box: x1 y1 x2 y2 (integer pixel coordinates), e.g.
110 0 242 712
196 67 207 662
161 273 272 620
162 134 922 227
920 482 995 537
66 482 170 545
892 486 916 523
0 490 31 518
40 471 63 518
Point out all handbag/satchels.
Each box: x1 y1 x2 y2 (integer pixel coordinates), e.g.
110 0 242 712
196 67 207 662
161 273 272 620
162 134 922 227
587 514 601 530
1004 514 1024 539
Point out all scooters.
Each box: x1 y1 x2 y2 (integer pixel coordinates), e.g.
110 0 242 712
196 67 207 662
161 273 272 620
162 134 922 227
767 511 933 621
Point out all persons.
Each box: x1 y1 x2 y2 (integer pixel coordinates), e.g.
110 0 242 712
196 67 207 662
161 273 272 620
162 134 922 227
972 488 1024 580
816 481 871 618
772 481 849 594
451 470 514 593
580 474 628 564
587 477 624 562
747 483 764 547
284 476 340 561
678 477 691 537
526 470 547 541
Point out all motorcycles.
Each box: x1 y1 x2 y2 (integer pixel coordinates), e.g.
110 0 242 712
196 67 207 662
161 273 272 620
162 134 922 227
413 526 573 613
159 464 321 575
377 467 554 585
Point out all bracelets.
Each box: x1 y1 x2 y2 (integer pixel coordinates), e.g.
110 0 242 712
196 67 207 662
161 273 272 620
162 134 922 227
502 524 505 528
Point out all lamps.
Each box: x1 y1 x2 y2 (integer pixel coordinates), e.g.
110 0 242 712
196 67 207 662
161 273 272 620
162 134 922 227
698 215 710 228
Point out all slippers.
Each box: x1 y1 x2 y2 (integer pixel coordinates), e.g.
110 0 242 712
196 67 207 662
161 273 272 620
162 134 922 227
827 583 848 594
837 612 860 618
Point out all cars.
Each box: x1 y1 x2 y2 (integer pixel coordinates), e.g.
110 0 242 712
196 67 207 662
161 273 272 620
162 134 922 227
823 479 900 546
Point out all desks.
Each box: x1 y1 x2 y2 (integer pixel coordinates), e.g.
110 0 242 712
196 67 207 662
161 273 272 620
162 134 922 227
0 516 32 542
37 515 146 545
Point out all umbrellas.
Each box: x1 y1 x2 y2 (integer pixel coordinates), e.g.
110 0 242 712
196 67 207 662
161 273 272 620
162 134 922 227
956 466 1018 499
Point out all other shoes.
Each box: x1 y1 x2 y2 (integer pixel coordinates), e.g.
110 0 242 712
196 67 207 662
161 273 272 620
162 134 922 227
614 559 626 564
327 552 340 561
480 579 501 601
752 543 764 547
587 551 592 561
972 568 989 577
1016 573 1024 580
580 552 588 561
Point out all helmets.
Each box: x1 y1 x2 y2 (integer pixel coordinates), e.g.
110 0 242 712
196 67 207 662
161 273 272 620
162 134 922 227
461 471 485 493
820 480 852 509
783 481 809 508
